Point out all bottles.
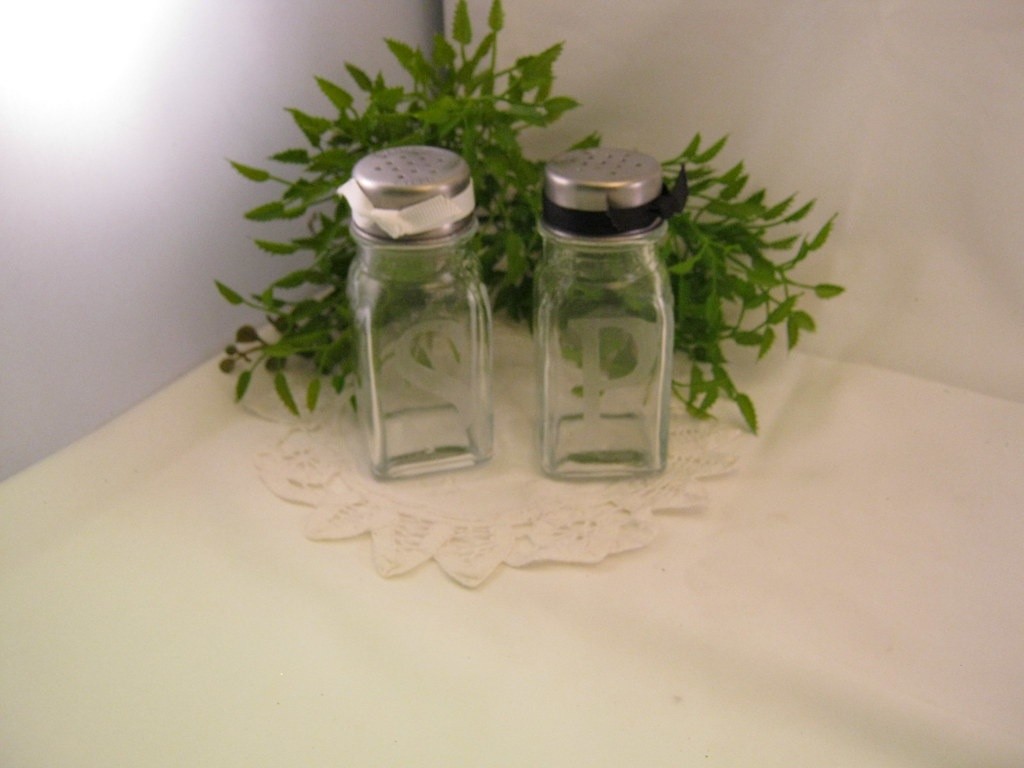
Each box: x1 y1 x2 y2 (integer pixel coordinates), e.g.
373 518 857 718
532 147 679 482
346 143 499 481
